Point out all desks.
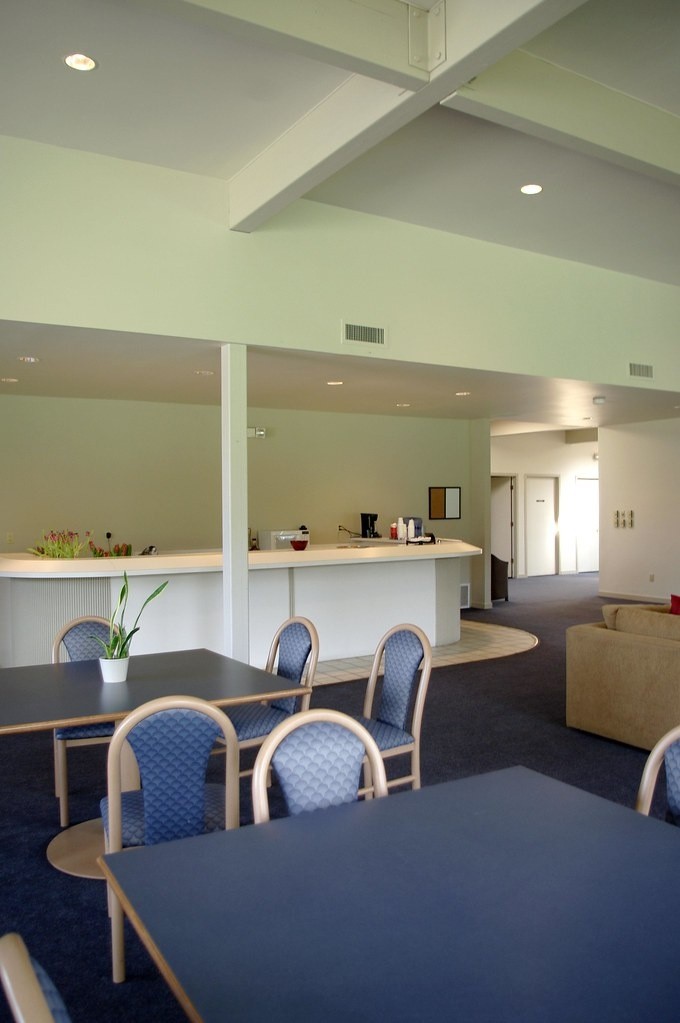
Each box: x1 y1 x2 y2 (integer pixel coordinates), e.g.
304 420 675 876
0 648 311 880
98 765 680 1023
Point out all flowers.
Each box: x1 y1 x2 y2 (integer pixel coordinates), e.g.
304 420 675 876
27 527 132 559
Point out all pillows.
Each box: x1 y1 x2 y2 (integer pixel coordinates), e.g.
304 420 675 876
602 594 680 640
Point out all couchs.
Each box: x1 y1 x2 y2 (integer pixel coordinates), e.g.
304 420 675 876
565 622 680 750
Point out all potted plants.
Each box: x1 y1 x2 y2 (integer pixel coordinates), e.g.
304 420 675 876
89 571 169 684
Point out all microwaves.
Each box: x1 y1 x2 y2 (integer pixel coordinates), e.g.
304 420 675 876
258 530 310 550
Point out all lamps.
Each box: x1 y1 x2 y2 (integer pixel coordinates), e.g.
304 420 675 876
247 427 266 439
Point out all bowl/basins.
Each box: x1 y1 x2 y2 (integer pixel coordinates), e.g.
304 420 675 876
291 541 308 550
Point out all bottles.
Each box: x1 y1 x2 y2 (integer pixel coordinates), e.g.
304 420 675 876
391 524 397 538
251 538 257 550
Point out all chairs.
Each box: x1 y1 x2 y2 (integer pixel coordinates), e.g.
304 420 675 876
351 623 432 802
50 616 128 828
97 694 240 983
251 710 387 825
1 932 72 1023
219 616 318 785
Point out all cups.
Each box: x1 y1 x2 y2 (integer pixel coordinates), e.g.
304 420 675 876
397 518 414 539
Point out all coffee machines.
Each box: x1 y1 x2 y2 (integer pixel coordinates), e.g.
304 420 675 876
361 513 378 538
